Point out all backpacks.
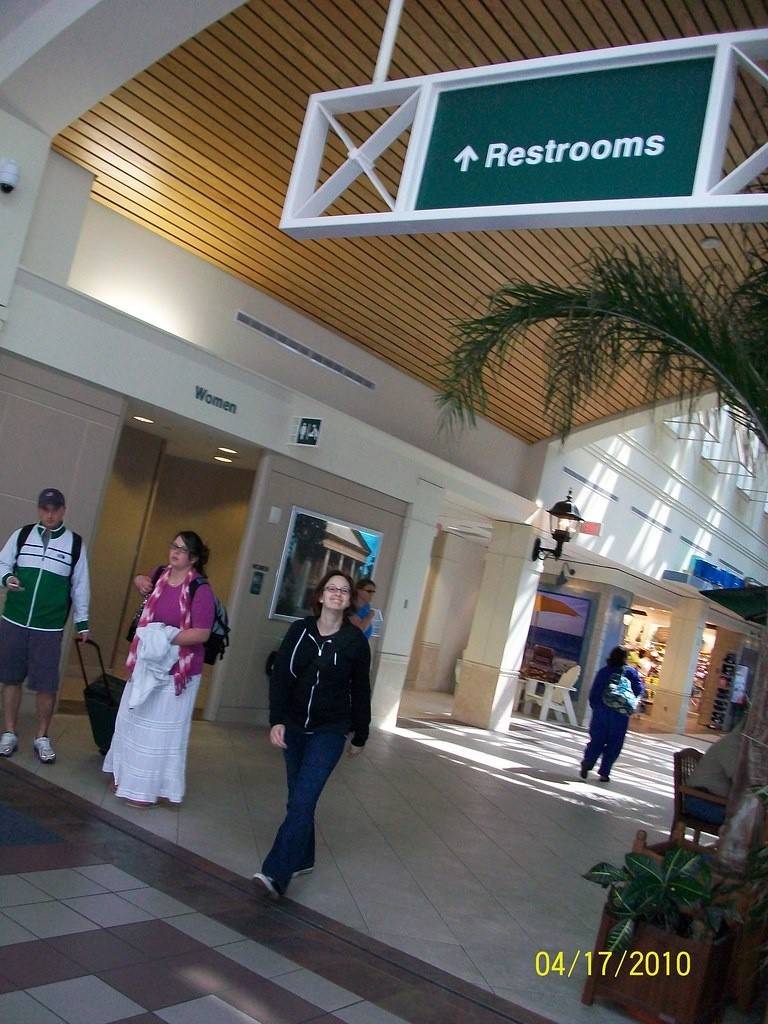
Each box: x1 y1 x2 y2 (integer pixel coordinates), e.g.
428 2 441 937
154 566 231 665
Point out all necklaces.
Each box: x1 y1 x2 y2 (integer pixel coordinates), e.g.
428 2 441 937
170 572 188 586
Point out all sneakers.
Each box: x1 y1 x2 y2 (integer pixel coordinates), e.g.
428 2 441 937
0 732 18 756
34 736 55 763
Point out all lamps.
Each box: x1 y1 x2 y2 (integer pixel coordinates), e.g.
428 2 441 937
619 605 648 626
533 487 588 563
556 563 576 586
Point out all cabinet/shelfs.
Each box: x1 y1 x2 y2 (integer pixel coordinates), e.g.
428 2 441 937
708 649 740 730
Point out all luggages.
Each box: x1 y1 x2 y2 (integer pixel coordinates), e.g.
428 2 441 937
75 637 127 759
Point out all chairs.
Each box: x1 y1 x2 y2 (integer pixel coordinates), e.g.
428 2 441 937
668 751 730 841
524 665 582 726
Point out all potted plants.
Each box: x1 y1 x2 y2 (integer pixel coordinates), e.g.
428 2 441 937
578 845 768 1024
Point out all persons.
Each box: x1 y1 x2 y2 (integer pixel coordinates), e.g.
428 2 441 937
0 487 92 763
579 644 643 782
683 713 768 860
251 569 371 900
633 649 652 681
351 578 377 641
102 527 218 808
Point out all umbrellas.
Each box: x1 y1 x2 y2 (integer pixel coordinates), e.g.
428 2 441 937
699 586 768 626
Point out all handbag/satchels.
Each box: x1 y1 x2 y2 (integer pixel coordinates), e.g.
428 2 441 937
602 665 639 716
126 592 151 642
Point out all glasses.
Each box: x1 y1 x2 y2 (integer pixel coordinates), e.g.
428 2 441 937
323 586 351 595
359 588 376 594
170 542 192 553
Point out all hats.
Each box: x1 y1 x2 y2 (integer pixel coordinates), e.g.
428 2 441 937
38 488 64 507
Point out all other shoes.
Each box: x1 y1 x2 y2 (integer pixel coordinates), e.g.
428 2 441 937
110 781 117 791
291 864 313 877
252 873 282 900
126 796 170 809
581 761 588 778
599 776 610 782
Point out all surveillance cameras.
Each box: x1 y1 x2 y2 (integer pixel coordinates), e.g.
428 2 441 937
0 164 20 193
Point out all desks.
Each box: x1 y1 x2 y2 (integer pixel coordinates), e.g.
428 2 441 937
512 679 526 711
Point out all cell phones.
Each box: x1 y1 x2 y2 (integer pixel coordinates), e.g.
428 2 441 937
9 583 20 589
